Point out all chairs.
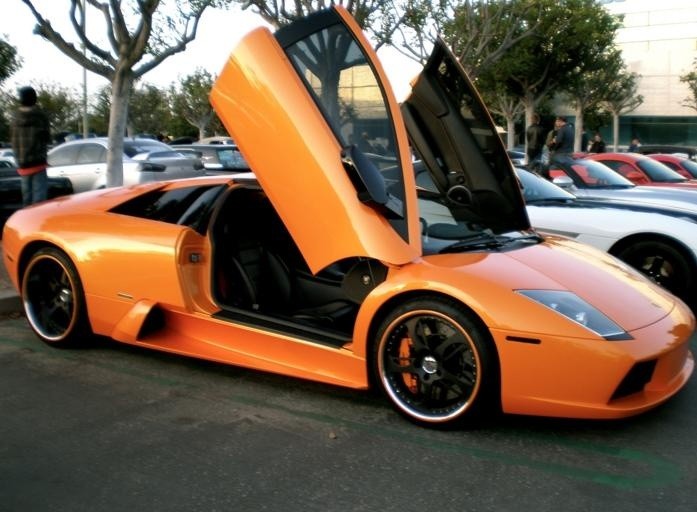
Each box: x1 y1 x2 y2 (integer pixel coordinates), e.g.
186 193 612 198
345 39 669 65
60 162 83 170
221 209 358 333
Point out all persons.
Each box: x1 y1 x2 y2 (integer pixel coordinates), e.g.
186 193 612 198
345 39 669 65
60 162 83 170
525 112 544 165
546 117 560 165
9 86 54 210
586 139 595 152
580 126 588 151
627 137 640 153
590 134 607 154
552 114 574 164
